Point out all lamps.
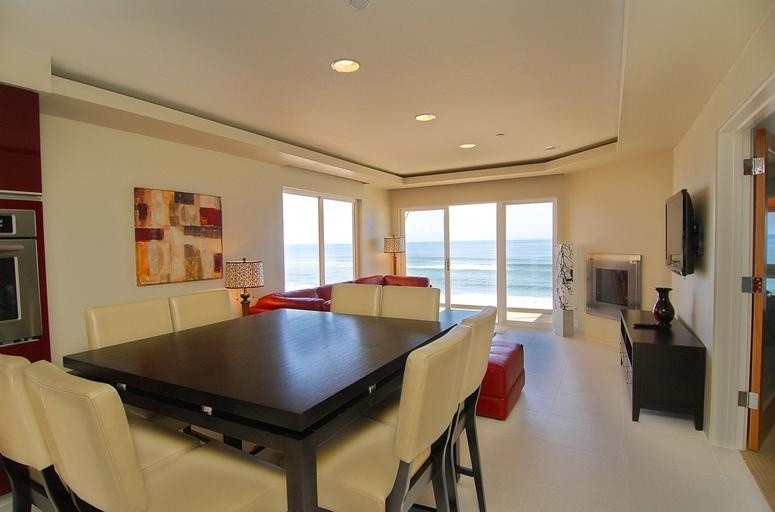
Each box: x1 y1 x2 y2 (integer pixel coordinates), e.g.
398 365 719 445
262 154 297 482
225 258 264 317
384 235 405 275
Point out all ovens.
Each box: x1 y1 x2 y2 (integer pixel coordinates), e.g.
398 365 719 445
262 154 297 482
0 208 43 348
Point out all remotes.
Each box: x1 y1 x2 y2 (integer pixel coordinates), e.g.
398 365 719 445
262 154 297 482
633 323 661 328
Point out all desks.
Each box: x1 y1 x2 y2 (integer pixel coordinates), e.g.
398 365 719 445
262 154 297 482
619 309 706 430
63 308 457 512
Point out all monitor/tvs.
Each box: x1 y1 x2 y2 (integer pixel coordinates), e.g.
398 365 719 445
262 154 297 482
664 188 703 276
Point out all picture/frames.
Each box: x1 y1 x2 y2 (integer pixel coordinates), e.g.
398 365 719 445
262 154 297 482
134 187 225 286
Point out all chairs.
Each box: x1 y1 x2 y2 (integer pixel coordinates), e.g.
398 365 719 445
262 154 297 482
0 353 80 512
169 289 232 332
380 285 440 322
369 306 497 511
330 283 382 317
84 298 173 351
316 323 471 511
23 359 289 512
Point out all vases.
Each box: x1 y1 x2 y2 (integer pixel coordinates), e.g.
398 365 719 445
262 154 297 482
651 288 674 327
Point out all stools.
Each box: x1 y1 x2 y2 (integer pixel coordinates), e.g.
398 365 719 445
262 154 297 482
476 341 525 420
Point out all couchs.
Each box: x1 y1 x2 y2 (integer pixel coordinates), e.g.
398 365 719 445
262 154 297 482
249 275 430 315
